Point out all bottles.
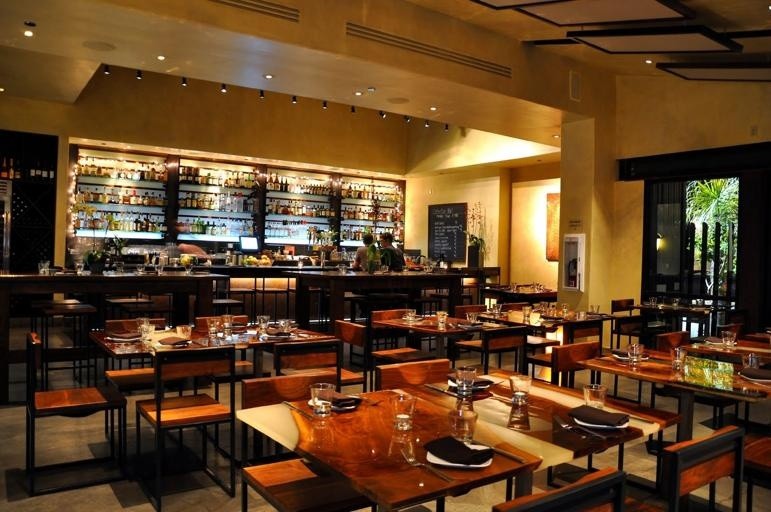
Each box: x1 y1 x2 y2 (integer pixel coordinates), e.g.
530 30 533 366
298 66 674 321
439 253 445 273
74 156 404 243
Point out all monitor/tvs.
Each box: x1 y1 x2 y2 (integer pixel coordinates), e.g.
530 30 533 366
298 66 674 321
239 234 261 253
403 250 420 265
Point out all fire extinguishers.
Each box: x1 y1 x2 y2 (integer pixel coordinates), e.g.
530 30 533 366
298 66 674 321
568 258 577 287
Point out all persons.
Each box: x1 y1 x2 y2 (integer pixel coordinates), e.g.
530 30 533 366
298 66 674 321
376 233 407 272
717 267 737 300
352 233 379 270
166 241 213 326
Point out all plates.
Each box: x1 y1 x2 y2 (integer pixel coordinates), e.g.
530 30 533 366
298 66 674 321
310 394 361 412
612 353 650 362
573 410 629 429
447 378 490 390
425 445 493 469
104 336 140 342
738 372 771 384
704 338 737 346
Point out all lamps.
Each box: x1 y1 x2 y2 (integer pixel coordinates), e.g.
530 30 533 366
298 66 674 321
405 117 410 122
444 124 449 132
221 83 227 94
379 112 386 119
136 71 142 81
258 91 265 99
351 106 356 113
292 96 297 104
181 78 188 87
425 121 430 128
323 102 327 109
104 65 111 75
472 1 770 82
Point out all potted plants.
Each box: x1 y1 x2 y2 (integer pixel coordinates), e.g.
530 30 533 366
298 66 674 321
464 231 484 267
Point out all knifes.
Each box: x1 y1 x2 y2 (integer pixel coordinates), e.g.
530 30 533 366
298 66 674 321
473 439 525 463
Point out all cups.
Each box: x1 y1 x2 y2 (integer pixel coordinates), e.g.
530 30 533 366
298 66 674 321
139 301 600 341
628 343 643 369
381 265 389 272
394 394 416 431
649 297 658 306
696 298 704 306
402 263 409 271
671 298 679 307
313 382 334 418
583 384 607 408
508 375 533 406
38 261 194 275
671 347 686 371
722 330 737 350
457 366 476 401
511 283 517 290
338 264 347 273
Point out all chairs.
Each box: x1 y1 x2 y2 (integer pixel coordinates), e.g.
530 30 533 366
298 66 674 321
492 468 628 512
240 370 374 510
628 426 744 512
24 331 127 497
136 345 236 511
709 428 770 512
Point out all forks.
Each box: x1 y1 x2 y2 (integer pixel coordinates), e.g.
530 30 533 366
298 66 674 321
401 448 452 482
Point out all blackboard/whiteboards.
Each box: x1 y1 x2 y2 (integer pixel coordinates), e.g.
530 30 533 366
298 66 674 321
428 202 466 263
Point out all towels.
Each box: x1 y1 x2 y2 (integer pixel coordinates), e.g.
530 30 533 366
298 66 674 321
422 436 496 466
